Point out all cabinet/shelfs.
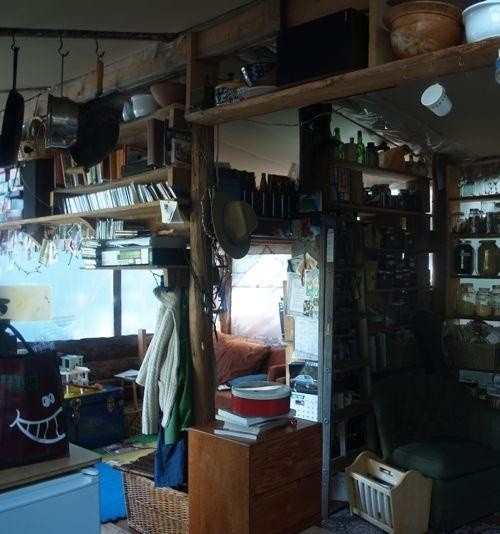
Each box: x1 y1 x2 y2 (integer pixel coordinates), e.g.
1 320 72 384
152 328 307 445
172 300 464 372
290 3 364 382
0 65 189 228
183 417 322 534
330 152 500 473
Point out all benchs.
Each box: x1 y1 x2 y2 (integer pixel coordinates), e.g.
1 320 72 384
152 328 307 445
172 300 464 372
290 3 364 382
215 347 288 414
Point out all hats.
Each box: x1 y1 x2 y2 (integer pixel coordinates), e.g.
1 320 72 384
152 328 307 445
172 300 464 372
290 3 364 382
210 191 259 261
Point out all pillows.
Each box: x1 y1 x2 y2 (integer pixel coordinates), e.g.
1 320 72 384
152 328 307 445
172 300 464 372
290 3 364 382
213 330 270 388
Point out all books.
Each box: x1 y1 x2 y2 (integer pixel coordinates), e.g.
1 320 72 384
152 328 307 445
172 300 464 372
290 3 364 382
223 417 290 434
368 329 419 373
218 407 296 425
331 167 366 462
48 112 193 269
216 413 251 429
214 425 260 440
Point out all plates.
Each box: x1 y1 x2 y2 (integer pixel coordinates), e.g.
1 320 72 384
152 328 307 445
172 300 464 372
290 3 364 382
236 86 280 100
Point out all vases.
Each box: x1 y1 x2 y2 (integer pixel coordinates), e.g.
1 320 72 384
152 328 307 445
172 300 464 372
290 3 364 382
382 1 499 58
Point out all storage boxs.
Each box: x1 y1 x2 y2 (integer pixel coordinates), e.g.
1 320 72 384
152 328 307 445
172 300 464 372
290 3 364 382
64 384 125 449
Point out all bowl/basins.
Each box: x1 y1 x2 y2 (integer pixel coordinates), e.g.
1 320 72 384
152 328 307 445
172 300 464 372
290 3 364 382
129 94 157 118
150 83 187 107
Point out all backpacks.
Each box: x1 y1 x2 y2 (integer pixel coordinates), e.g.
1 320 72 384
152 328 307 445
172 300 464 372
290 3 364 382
0 322 71 471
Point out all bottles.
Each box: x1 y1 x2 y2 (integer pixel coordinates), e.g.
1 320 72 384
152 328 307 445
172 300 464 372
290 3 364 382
243 170 297 219
449 208 500 344
333 125 429 372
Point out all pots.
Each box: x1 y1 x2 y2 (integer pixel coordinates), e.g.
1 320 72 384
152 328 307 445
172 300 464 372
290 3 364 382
0 47 124 170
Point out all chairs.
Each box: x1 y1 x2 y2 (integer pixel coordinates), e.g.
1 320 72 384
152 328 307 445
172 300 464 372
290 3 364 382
371 364 500 533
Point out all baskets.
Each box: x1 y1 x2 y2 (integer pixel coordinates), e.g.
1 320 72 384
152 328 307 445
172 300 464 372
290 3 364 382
111 447 189 533
